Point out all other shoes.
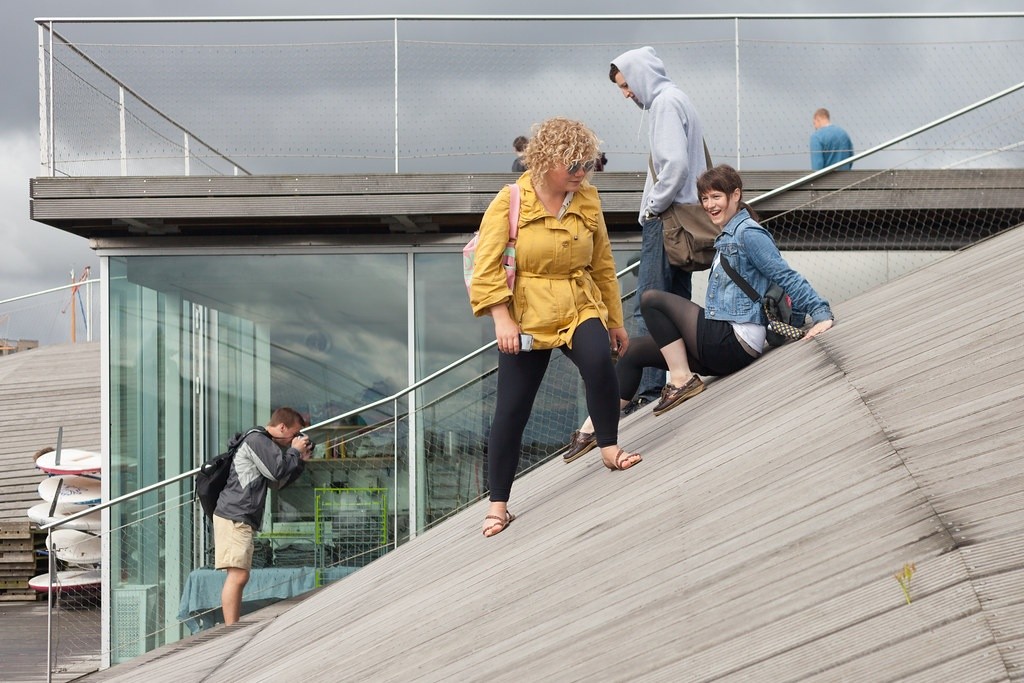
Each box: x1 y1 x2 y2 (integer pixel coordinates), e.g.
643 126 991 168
624 385 662 415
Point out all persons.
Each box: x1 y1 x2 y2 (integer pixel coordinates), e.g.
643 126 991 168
197 409 316 627
563 165 834 462
812 108 854 171
609 46 719 416
463 117 642 537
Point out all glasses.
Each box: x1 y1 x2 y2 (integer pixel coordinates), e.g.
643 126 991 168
559 159 594 175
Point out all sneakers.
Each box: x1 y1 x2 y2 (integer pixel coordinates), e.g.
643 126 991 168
652 373 706 416
563 428 598 463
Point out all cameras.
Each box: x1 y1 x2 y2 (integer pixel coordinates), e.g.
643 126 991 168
293 431 316 451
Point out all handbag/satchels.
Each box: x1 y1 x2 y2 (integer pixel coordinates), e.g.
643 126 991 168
757 283 807 349
661 205 722 271
462 184 521 316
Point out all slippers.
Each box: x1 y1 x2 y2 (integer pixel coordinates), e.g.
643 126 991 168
483 510 515 538
601 449 642 471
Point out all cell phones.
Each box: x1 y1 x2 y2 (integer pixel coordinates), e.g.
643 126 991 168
497 333 534 352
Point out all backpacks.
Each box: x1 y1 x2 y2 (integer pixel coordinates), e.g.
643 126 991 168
197 429 274 526
